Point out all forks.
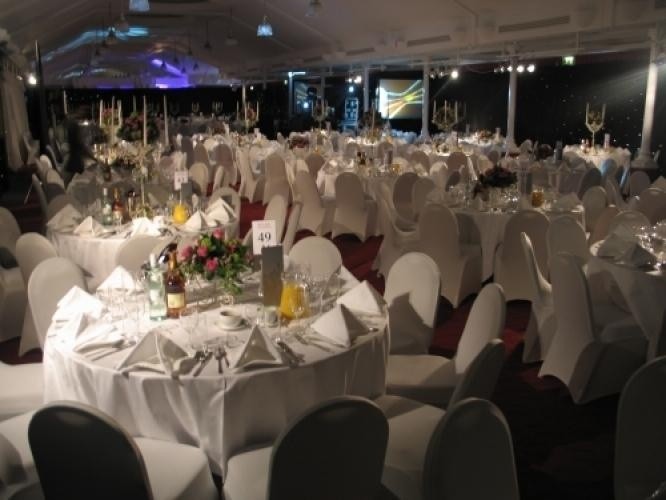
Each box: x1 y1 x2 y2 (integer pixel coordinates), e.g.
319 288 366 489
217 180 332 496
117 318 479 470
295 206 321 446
293 333 331 352
214 345 229 374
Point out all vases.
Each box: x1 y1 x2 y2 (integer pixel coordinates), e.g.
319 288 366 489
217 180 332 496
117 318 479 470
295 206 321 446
221 290 238 308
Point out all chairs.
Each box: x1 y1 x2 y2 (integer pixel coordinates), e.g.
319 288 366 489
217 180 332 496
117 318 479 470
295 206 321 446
548 217 593 265
262 153 294 207
377 179 418 232
425 395 521 500
548 253 651 403
429 160 449 186
331 172 378 243
341 142 363 165
213 144 238 186
29 398 217 499
384 281 507 405
15 233 60 299
412 174 436 220
140 183 176 210
419 203 483 308
286 236 343 284
226 397 392 500
380 253 441 354
582 185 609 231
521 230 557 365
587 205 621 247
207 187 241 238
188 163 209 195
493 212 552 305
292 170 336 237
0 205 24 267
635 188 666 224
393 169 423 227
0 262 30 346
115 235 164 279
29 257 88 356
235 146 266 205
377 198 418 275
411 151 431 176
364 339 506 498
44 194 83 223
0 402 50 499
597 211 658 270
376 143 394 166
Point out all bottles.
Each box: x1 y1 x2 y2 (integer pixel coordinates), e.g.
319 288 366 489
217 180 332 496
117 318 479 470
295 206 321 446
126 190 134 220
165 251 186 318
585 139 590 153
100 187 111 225
580 139 586 154
111 187 124 225
145 253 168 320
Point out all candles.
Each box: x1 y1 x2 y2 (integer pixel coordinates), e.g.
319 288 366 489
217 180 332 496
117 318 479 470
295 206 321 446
256 100 259 120
432 99 436 123
601 102 605 124
321 99 325 118
585 102 590 126
311 100 314 120
110 97 115 128
454 101 458 124
98 99 103 129
443 99 448 125
118 100 124 129
247 101 250 119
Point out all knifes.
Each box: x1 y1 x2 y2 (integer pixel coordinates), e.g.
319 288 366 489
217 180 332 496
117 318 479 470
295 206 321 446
277 341 299 361
192 351 213 377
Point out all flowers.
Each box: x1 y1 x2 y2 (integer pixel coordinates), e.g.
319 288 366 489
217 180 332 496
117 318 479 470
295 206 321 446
181 222 248 294
479 162 519 189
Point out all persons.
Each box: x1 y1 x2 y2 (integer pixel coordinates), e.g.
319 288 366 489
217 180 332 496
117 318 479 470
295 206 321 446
37 123 70 182
62 124 96 186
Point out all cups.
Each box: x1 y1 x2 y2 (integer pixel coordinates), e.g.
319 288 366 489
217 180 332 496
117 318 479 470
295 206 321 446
219 309 241 325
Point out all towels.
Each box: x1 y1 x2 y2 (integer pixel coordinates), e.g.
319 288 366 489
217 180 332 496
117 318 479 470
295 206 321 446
337 280 387 314
184 211 208 230
131 218 161 238
52 214 77 231
74 216 108 236
597 234 628 259
556 195 576 209
54 287 104 323
72 325 135 364
207 199 237 216
313 304 368 347
233 324 284 373
47 204 80 225
620 243 656 268
566 192 580 205
97 267 144 293
210 205 234 223
117 328 193 377
608 223 636 241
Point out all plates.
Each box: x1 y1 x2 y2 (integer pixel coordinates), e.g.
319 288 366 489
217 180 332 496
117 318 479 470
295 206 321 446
213 322 246 330
